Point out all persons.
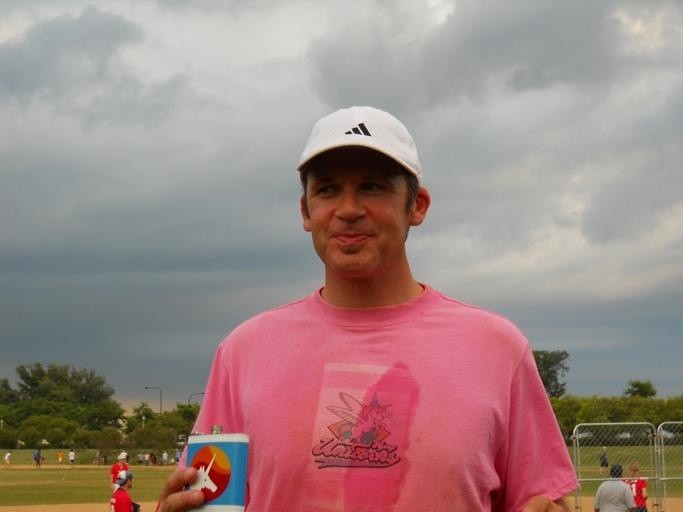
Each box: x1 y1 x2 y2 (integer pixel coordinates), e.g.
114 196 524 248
125 449 182 467
599 445 609 474
95 450 101 465
593 465 637 512
110 450 130 491
110 470 140 512
624 462 649 512
155 106 580 512
31 449 78 469
4 451 12 464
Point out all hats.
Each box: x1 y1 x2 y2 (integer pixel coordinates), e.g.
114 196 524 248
116 454 126 461
114 470 132 485
295 105 423 189
608 464 624 478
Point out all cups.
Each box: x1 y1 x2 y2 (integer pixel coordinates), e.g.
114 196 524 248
185 433 250 511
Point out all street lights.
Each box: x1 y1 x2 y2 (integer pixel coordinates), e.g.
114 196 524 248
185 391 206 404
141 416 146 430
143 384 164 418
